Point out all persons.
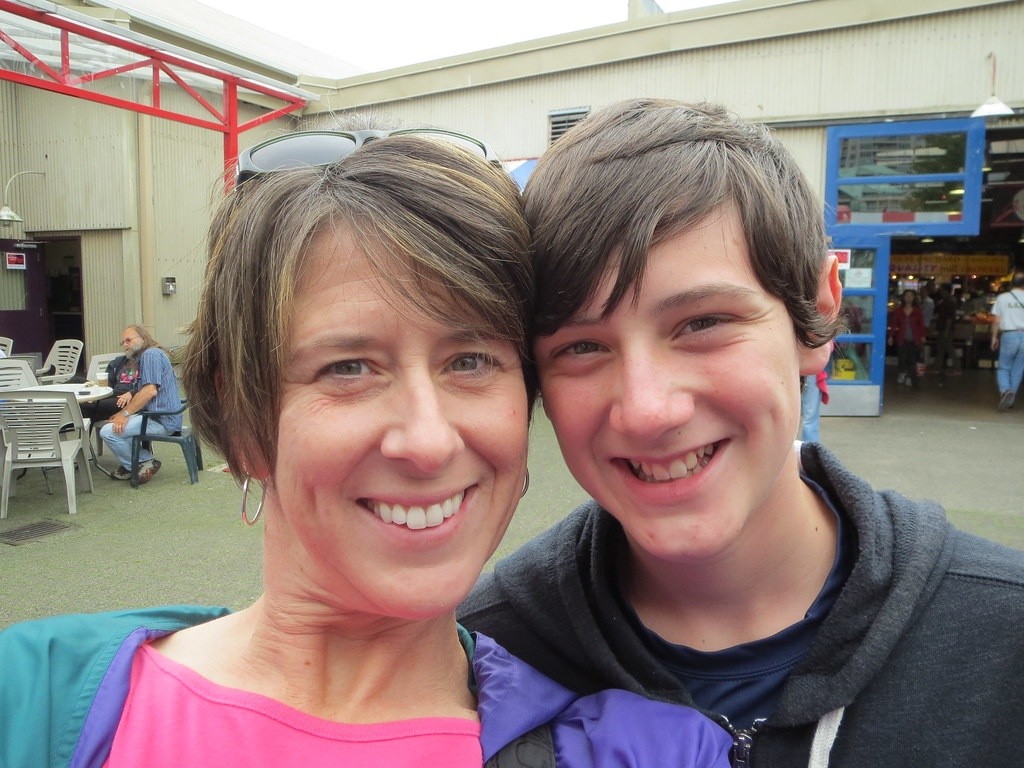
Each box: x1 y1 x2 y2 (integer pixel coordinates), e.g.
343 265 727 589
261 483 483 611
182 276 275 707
457 95 1024 768
78 353 139 439
890 272 1024 410
0 128 735 768
100 322 181 486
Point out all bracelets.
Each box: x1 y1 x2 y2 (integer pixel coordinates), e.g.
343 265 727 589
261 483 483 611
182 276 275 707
123 410 129 417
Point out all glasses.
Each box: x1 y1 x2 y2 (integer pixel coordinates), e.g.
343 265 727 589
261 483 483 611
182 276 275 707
120 336 139 345
236 127 505 188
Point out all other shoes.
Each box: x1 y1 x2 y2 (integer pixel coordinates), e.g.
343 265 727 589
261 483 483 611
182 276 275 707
905 378 912 386
897 373 906 383
998 390 1014 411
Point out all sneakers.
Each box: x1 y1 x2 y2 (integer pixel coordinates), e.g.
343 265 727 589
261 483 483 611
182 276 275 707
137 459 161 485
111 465 133 480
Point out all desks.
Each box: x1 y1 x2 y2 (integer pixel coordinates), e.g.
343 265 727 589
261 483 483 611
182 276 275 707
10 383 114 495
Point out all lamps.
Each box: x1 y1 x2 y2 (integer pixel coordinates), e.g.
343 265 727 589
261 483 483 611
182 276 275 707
969 52 1015 118
0 171 45 227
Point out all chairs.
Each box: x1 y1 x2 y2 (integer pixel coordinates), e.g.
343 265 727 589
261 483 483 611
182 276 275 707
0 337 13 357
0 359 38 487
86 353 126 386
0 391 93 519
37 339 84 386
0 356 42 386
131 398 203 489
